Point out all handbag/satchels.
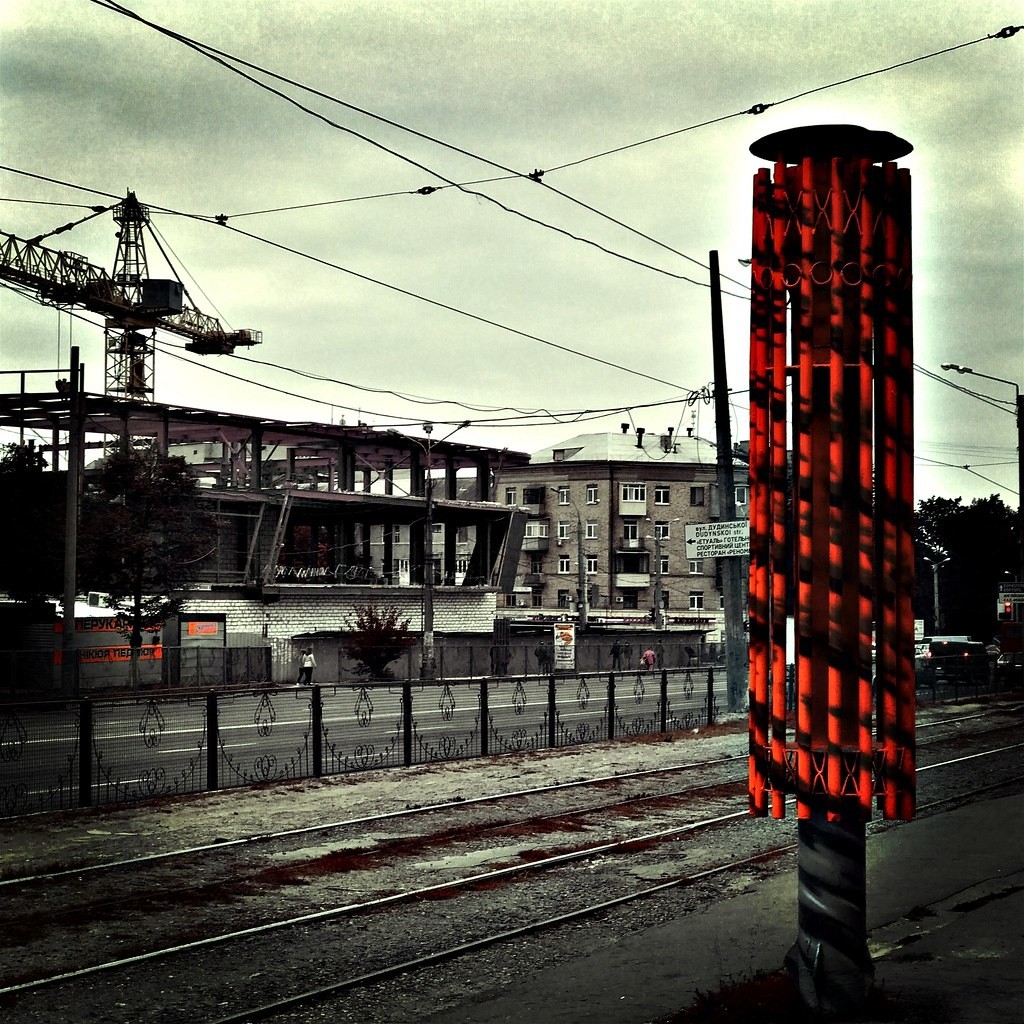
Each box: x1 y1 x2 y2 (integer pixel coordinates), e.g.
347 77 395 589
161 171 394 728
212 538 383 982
653 655 656 663
640 659 645 666
304 661 312 667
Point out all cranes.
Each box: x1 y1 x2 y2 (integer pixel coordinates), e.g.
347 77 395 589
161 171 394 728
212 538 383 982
0 188 262 469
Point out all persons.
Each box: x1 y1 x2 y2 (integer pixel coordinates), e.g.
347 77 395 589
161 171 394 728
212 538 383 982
608 639 633 671
639 638 665 670
535 641 555 675
296 648 317 686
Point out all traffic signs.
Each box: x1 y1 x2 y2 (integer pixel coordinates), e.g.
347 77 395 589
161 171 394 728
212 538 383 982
686 519 750 560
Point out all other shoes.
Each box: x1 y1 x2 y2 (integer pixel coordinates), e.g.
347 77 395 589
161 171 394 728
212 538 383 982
296 683 300 686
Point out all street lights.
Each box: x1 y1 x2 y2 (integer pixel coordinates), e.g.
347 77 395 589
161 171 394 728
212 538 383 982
549 487 588 631
923 556 950 636
646 518 680 630
941 363 1024 584
386 419 475 681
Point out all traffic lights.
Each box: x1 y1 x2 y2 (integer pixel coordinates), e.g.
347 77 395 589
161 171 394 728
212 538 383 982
1004 599 1011 620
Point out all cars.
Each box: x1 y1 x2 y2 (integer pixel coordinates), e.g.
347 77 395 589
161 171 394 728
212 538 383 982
909 635 1024 686
